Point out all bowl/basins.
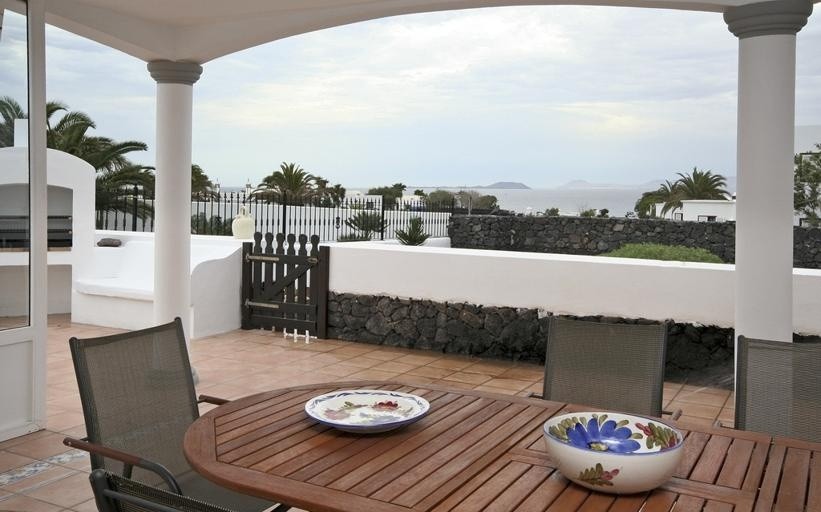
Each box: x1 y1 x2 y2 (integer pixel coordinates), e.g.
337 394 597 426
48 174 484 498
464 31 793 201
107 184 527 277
543 409 686 495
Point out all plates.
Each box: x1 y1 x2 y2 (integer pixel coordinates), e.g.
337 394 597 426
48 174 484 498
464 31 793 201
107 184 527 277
303 389 429 434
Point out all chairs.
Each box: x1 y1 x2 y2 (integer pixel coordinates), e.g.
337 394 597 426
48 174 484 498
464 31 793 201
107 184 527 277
529 319 683 420
62 317 290 511
734 335 818 442
